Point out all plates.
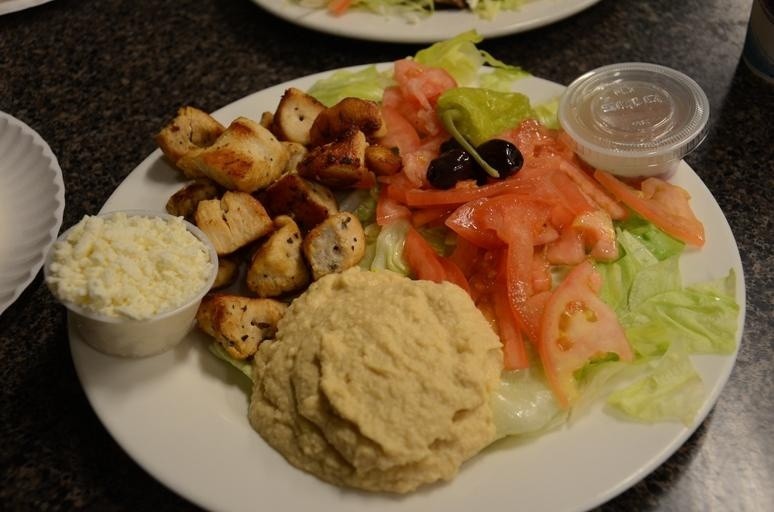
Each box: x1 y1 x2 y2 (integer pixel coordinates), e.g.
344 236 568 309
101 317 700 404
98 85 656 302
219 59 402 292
70 61 748 512
0 113 67 311
260 2 600 45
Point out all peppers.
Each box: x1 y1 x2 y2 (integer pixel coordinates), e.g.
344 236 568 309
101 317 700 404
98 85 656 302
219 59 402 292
435 87 535 179
